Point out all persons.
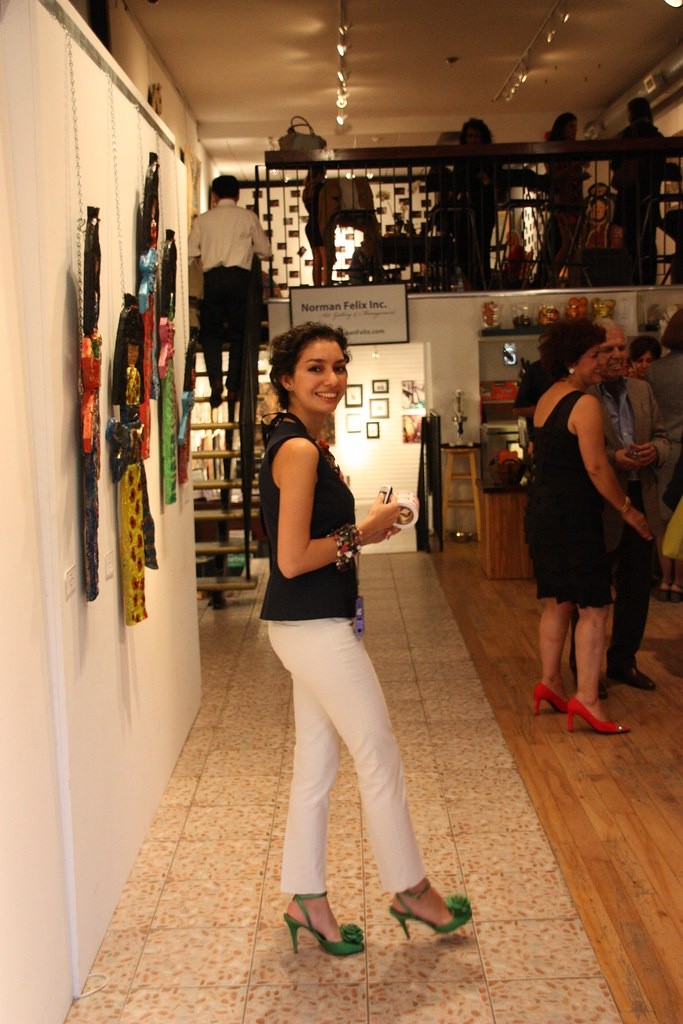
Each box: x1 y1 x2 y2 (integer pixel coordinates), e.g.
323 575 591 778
257 320 472 958
537 111 591 280
452 118 507 269
188 175 271 406
523 317 655 738
607 98 667 287
523 309 683 736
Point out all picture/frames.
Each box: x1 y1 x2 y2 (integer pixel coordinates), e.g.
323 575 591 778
369 397 391 417
343 382 362 412
366 421 381 440
347 412 362 434
373 378 390 393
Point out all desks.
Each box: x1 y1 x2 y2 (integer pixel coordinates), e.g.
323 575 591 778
354 234 467 304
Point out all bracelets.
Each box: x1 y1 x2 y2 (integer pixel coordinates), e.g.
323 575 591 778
326 522 363 572
618 495 631 514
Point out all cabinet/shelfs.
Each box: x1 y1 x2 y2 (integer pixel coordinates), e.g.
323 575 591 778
471 325 613 580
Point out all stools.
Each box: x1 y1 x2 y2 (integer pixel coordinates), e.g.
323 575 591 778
442 441 483 542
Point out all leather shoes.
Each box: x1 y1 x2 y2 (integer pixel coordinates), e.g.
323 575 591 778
573 675 608 699
606 664 656 691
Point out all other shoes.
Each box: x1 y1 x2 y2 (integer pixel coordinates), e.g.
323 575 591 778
209 379 224 409
227 389 237 402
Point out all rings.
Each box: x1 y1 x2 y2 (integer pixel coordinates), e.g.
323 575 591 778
641 522 647 529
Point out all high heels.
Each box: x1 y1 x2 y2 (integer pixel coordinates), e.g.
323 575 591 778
283 893 365 956
567 695 631 734
388 882 473 941
533 682 567 716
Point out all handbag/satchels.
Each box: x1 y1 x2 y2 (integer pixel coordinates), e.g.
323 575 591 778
278 116 327 150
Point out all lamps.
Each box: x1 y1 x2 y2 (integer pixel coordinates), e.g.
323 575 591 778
337 23 353 128
505 10 569 101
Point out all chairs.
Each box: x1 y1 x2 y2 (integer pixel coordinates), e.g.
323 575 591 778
493 168 597 290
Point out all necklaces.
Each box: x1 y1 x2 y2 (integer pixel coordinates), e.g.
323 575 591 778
308 434 344 481
